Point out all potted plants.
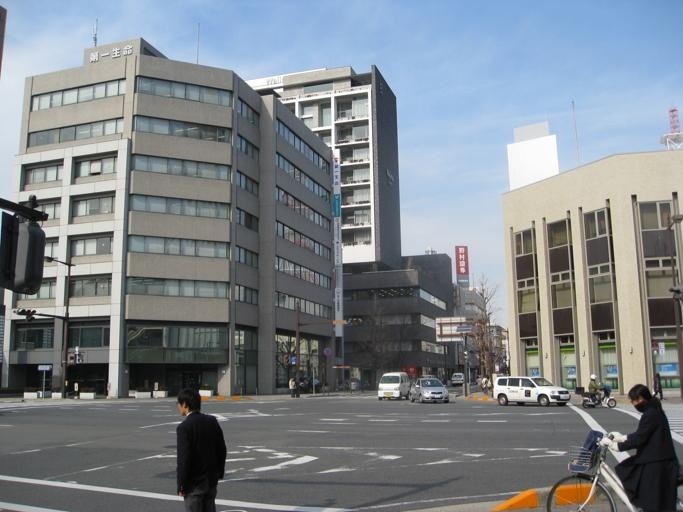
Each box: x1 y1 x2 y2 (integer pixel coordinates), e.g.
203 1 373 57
22 384 96 400
197 384 214 397
128 387 168 399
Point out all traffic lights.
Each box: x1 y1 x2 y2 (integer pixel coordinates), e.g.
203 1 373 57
346 317 362 327
666 285 682 303
16 308 36 316
4 220 46 297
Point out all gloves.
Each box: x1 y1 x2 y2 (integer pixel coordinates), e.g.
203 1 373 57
600 437 618 451
608 431 627 443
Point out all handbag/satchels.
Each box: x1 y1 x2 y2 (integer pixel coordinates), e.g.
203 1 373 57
578 429 603 464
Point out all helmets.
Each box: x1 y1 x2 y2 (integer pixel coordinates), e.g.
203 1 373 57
590 374 597 379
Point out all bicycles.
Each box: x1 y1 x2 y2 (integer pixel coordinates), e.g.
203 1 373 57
545 426 682 512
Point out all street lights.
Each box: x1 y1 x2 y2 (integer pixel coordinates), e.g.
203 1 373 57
665 212 682 399
44 255 75 399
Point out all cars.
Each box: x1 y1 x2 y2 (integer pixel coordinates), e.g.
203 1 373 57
408 376 449 404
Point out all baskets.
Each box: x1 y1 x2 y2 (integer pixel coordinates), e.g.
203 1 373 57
567 444 598 474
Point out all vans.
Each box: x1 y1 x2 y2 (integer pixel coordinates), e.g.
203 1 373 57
451 373 464 386
492 376 571 406
376 370 409 401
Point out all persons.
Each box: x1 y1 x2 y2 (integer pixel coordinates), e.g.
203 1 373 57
289 377 297 398
476 375 493 392
175 389 227 512
588 374 605 403
598 384 679 512
653 372 664 400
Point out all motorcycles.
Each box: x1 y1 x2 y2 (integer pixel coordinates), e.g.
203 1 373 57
575 383 616 409
